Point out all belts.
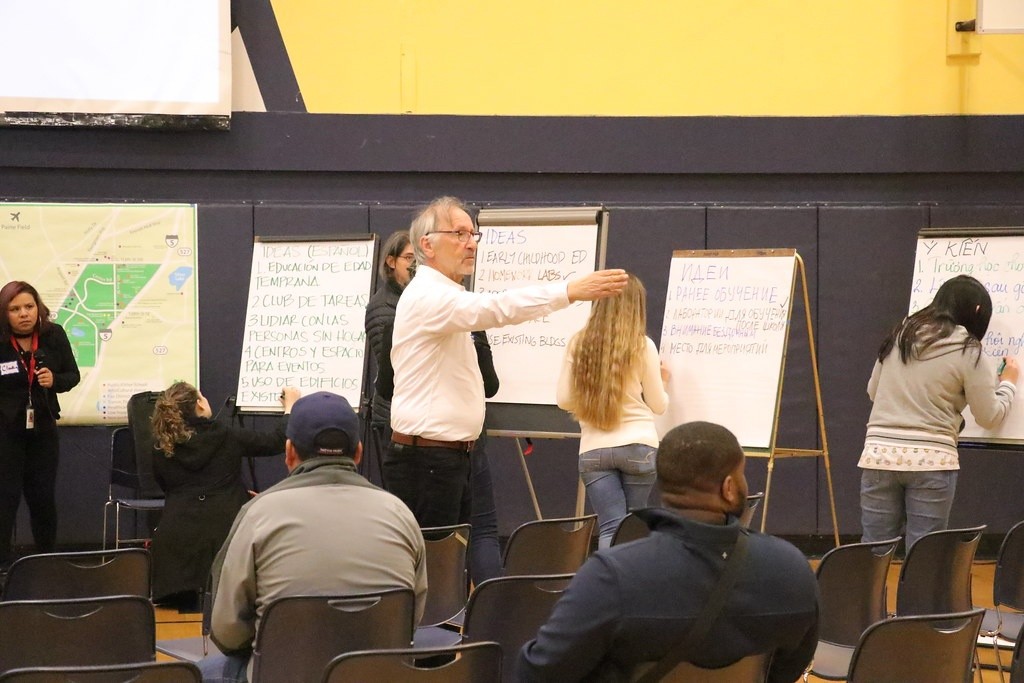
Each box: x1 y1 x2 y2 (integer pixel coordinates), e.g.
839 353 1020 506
391 432 476 453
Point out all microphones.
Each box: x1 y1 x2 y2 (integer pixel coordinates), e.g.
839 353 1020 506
34 350 49 396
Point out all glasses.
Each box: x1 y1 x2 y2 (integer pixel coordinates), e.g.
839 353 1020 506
426 229 482 243
396 254 414 264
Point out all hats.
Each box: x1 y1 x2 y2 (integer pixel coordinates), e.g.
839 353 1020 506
284 392 360 456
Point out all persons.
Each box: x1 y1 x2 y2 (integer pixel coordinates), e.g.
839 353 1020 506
856 275 1021 554
517 422 821 683
391 196 630 542
363 231 502 584
211 391 428 683
557 273 672 550
150 382 301 612
0 281 81 572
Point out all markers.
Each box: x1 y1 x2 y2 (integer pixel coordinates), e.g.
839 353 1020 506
997 362 1007 376
280 395 285 398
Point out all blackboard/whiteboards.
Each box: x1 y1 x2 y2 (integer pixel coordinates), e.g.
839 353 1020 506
469 207 609 439
236 233 380 418
909 226 1024 445
654 249 797 457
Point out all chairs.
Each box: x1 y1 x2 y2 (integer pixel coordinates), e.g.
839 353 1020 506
0 391 1024 683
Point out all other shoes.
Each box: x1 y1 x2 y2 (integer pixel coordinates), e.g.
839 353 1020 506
151 596 171 606
0 566 10 574
